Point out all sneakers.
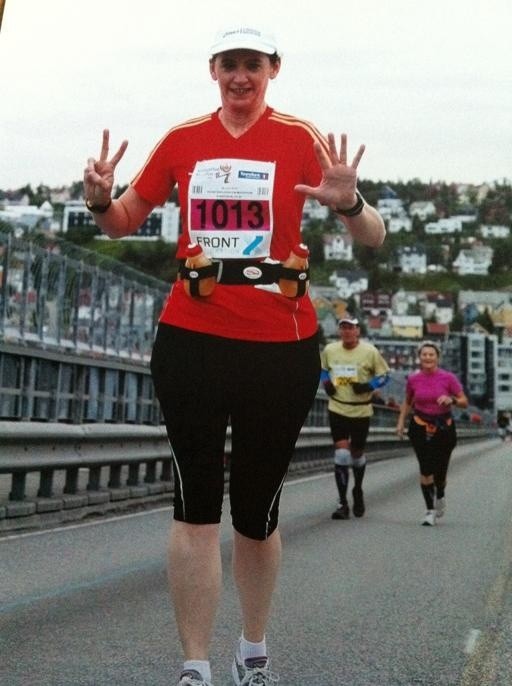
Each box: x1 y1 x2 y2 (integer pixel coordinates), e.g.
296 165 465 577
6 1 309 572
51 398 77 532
331 501 349 520
231 645 279 686
434 494 446 518
352 487 365 517
178 667 212 686
422 508 436 526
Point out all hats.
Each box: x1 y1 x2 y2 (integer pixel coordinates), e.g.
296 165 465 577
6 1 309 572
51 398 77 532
336 313 359 329
207 24 281 57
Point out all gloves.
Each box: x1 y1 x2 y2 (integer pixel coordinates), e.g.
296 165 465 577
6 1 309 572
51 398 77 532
323 380 336 396
350 381 373 393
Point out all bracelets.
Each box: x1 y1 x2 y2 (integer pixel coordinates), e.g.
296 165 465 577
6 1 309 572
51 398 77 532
451 395 457 406
86 196 113 215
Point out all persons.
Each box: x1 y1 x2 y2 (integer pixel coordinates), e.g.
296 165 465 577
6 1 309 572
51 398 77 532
394 338 469 525
319 314 394 522
82 21 386 686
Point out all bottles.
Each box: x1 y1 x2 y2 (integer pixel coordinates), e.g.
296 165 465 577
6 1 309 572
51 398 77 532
279 242 312 299
182 243 216 300
